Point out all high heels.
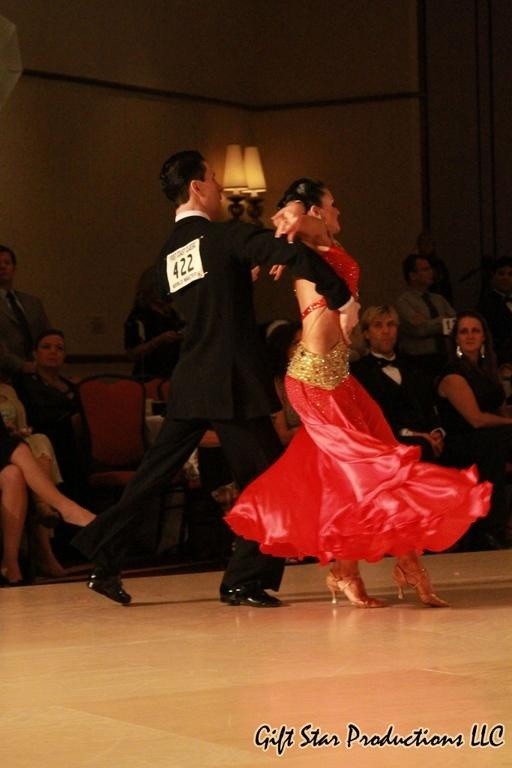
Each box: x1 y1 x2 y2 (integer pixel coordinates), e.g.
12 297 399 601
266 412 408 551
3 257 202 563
325 564 386 609
389 561 452 609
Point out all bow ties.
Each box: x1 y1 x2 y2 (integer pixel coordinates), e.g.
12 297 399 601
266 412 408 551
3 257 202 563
377 357 400 368
503 296 512 303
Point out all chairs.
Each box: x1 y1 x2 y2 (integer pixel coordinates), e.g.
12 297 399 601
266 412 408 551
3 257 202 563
61 369 227 558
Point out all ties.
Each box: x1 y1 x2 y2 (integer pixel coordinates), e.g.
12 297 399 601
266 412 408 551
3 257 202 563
421 292 448 361
5 290 34 363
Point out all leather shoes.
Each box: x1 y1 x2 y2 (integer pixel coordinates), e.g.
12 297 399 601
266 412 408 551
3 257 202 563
219 578 283 608
86 566 132 605
61 514 97 536
0 571 27 587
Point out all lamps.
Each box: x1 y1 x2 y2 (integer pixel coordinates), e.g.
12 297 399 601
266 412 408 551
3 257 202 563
221 143 271 225
